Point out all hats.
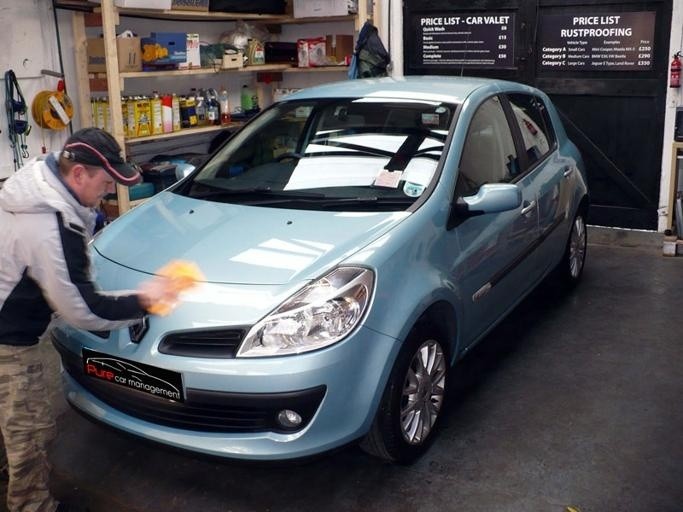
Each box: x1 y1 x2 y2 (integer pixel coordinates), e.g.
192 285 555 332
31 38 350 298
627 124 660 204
61 127 144 186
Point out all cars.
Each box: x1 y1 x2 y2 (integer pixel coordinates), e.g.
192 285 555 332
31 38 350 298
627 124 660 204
51 76 591 465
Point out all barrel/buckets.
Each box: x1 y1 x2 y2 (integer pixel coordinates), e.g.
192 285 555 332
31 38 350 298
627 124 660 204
241 84 258 111
244 37 267 65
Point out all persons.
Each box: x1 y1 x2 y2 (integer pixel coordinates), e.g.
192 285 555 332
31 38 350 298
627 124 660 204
0 127 162 512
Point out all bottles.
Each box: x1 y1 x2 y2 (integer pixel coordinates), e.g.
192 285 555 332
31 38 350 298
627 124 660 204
220 90 230 125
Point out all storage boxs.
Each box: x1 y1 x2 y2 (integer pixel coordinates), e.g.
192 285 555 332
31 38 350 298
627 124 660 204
83 34 140 74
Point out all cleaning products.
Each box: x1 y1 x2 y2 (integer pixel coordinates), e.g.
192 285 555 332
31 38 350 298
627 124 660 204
180 86 230 126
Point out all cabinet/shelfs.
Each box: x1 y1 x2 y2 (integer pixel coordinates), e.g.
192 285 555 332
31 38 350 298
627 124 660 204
72 1 380 222
